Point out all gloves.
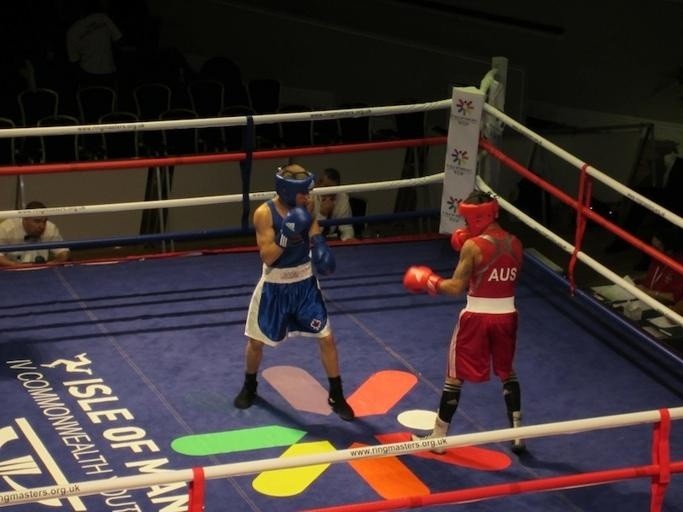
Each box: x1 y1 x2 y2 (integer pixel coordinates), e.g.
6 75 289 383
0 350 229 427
275 208 312 248
403 266 441 297
310 233 335 275
451 228 471 252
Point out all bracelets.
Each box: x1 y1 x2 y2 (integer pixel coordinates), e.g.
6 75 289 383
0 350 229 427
655 291 659 297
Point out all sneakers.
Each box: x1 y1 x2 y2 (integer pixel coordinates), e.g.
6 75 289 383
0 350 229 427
411 433 447 454
235 381 257 408
512 439 525 452
328 392 354 420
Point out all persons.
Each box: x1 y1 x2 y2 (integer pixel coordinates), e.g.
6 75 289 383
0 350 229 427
633 229 682 301
0 199 71 269
310 166 354 246
231 161 355 422
401 186 527 456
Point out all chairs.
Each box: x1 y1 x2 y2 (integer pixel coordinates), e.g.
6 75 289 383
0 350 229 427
0 55 430 164
349 197 369 238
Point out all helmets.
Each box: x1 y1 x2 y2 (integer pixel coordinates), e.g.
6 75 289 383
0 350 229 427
459 194 499 234
275 164 315 206
